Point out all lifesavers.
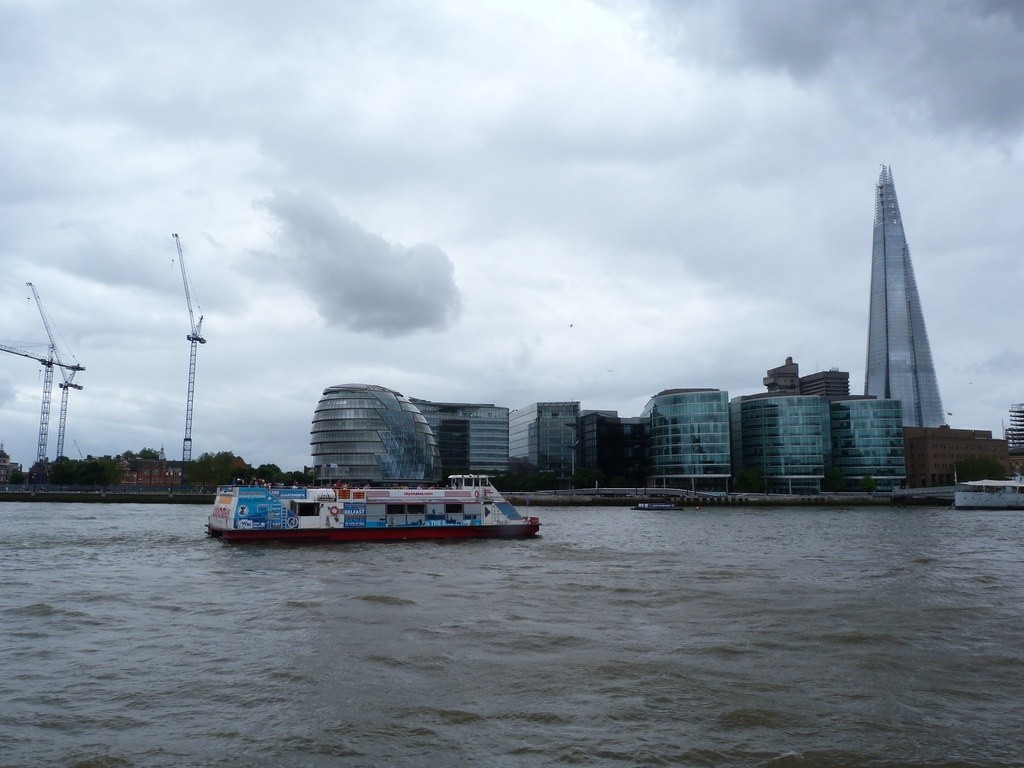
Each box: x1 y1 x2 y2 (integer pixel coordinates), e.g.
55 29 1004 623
475 490 479 498
962 496 1021 501
287 517 298 528
213 507 231 519
330 506 339 515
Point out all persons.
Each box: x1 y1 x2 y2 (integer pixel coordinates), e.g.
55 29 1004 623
235 474 460 490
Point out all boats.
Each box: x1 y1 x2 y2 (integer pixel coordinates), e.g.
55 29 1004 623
204 474 542 541
630 502 685 510
954 479 1024 510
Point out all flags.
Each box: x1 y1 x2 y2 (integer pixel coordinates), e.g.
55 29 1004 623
948 412 952 415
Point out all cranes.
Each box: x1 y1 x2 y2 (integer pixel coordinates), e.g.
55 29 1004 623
171 233 208 493
1 339 87 462
25 282 83 463
